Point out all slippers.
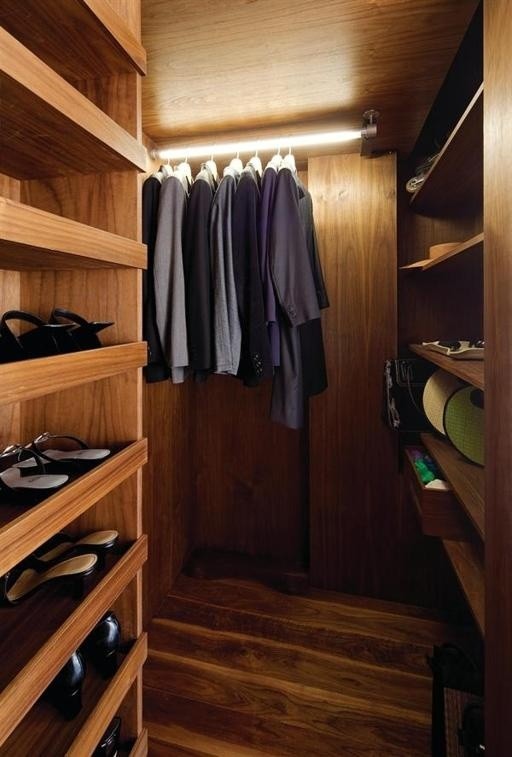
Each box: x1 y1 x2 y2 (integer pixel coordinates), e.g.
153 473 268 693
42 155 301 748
0 432 111 508
0 529 120 607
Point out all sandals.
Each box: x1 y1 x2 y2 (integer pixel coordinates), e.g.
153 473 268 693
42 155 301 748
0 307 115 364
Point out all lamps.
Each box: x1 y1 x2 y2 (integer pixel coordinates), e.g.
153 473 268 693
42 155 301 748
150 105 382 161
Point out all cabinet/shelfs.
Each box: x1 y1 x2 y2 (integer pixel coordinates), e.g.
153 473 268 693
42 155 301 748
0 0 150 757
397 0 512 757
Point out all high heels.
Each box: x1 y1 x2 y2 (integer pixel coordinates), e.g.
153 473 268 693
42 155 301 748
32 611 121 722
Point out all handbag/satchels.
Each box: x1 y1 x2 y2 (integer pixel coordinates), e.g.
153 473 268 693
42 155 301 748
381 358 485 468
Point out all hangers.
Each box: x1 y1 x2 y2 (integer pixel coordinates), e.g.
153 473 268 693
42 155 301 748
164 147 296 170
